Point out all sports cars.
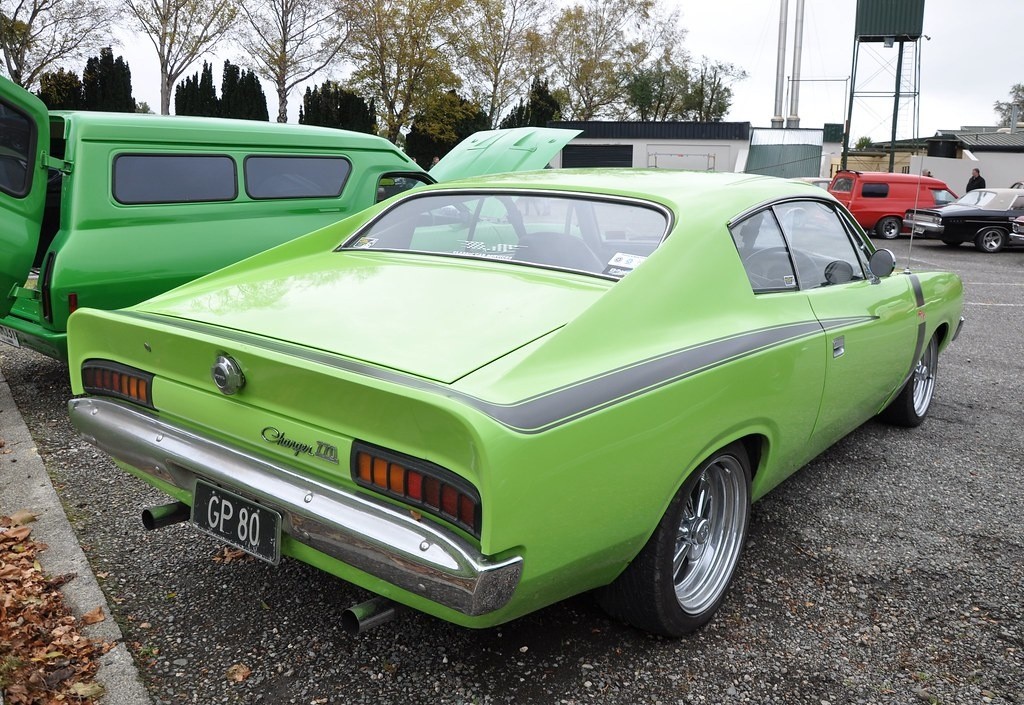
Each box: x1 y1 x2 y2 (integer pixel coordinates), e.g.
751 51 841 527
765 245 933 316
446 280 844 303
68 177 964 638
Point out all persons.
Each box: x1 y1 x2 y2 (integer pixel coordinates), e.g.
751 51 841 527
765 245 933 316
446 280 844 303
430 157 439 169
966 168 985 193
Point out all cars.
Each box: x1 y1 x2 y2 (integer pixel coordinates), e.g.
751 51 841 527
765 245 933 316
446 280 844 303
777 178 831 231
904 187 1024 254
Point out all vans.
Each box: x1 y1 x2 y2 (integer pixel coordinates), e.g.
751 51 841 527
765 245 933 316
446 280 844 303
0 76 586 366
827 168 961 240
